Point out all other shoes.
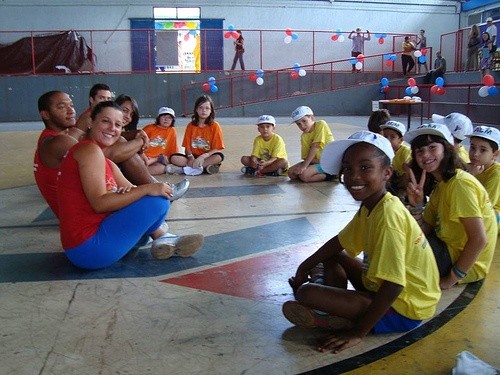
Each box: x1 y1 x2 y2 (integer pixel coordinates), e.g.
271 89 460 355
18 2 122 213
206 165 218 174
151 232 203 259
265 168 282 176
282 300 347 331
241 166 258 174
166 164 185 174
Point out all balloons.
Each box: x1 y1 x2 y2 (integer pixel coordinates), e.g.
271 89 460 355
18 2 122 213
225 24 344 44
349 54 364 70
431 77 446 94
478 75 497 97
414 48 427 63
375 33 387 44
382 78 390 93
202 63 306 94
384 53 397 66
405 78 419 95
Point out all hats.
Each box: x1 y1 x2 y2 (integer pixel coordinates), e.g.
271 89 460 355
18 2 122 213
288 106 313 123
464 126 500 150
432 113 473 140
320 131 395 174
380 121 406 137
406 123 454 146
157 107 175 118
256 115 275 127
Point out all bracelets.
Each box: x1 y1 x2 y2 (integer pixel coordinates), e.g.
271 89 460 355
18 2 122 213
452 262 466 279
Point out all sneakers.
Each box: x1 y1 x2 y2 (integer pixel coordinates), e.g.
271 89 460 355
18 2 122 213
164 179 190 203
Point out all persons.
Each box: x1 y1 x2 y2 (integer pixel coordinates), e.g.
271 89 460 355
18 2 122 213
143 95 225 176
288 106 339 182
368 109 498 289
241 115 290 175
414 30 429 75
348 27 370 74
422 51 446 84
231 30 245 70
401 36 416 76
282 130 441 353
34 83 169 244
465 17 497 83
58 101 204 269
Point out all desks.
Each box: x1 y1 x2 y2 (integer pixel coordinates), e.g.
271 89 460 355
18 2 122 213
378 97 428 128
